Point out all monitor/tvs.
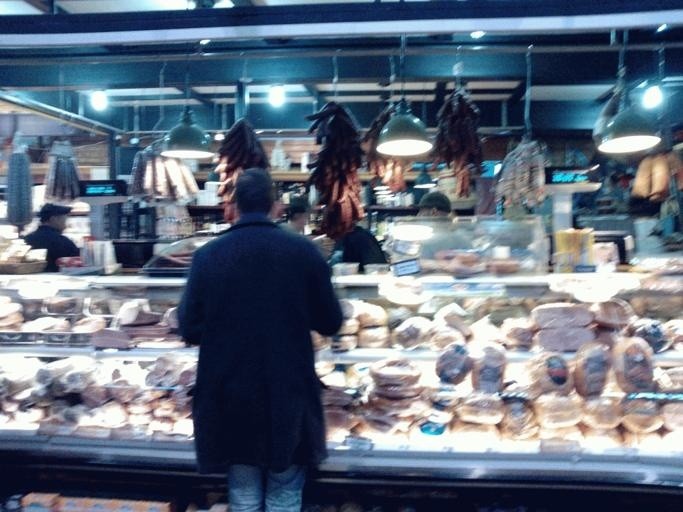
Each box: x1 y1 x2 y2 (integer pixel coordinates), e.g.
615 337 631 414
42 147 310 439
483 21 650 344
576 215 638 263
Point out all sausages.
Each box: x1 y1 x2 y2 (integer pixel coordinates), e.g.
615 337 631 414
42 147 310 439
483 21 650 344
361 98 416 196
304 102 370 239
430 88 484 200
215 117 273 228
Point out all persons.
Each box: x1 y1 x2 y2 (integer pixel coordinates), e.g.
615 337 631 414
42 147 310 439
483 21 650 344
322 201 384 263
177 165 343 511
415 191 451 218
22 202 78 271
279 199 310 235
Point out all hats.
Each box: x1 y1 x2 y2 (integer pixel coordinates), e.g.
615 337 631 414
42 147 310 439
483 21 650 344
38 204 72 222
409 191 454 216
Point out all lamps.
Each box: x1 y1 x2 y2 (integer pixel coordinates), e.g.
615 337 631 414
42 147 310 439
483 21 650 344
373 33 436 155
596 43 663 155
155 42 215 160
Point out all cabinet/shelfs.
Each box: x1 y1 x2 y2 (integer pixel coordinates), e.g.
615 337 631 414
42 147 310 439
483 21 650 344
383 217 549 275
0 263 683 480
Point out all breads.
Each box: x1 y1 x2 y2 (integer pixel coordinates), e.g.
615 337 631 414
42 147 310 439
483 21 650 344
0 241 683 457
